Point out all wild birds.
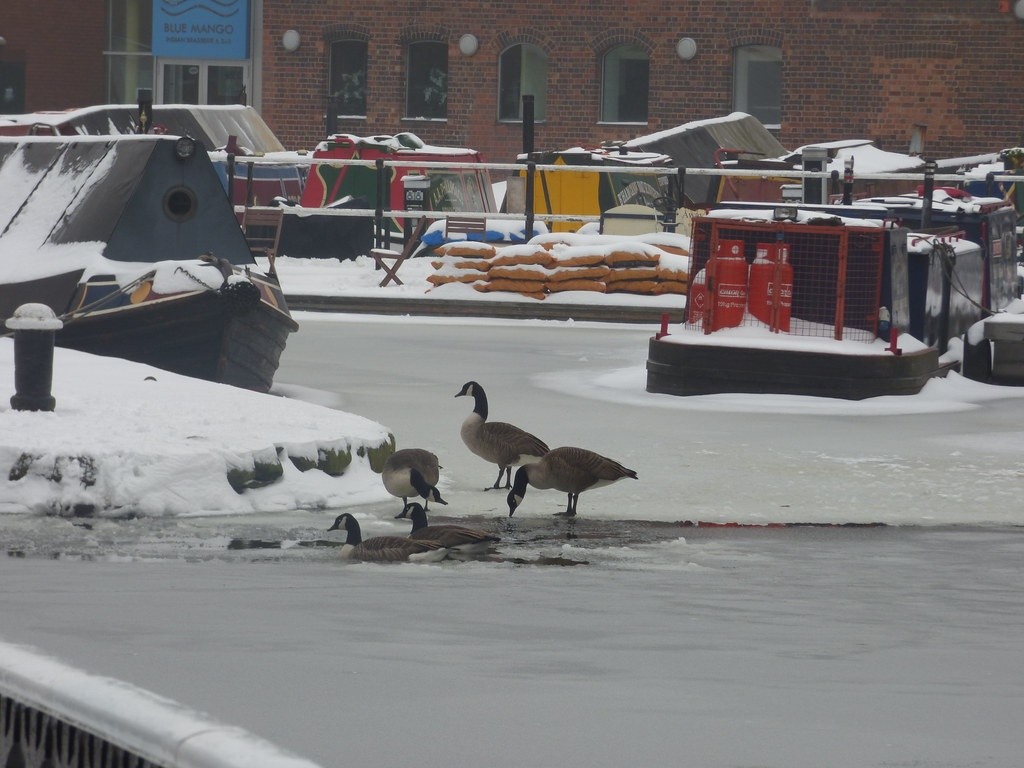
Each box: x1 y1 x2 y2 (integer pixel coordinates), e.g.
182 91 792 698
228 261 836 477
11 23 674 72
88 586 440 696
380 448 449 512
454 381 551 491
506 446 639 518
326 513 461 564
394 503 502 554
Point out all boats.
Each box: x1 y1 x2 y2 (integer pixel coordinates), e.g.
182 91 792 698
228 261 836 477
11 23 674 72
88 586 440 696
645 158 1018 404
0 88 301 394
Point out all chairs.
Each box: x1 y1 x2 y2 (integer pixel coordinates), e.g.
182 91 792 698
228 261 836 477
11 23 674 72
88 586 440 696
370 212 426 286
444 214 488 245
240 205 285 284
